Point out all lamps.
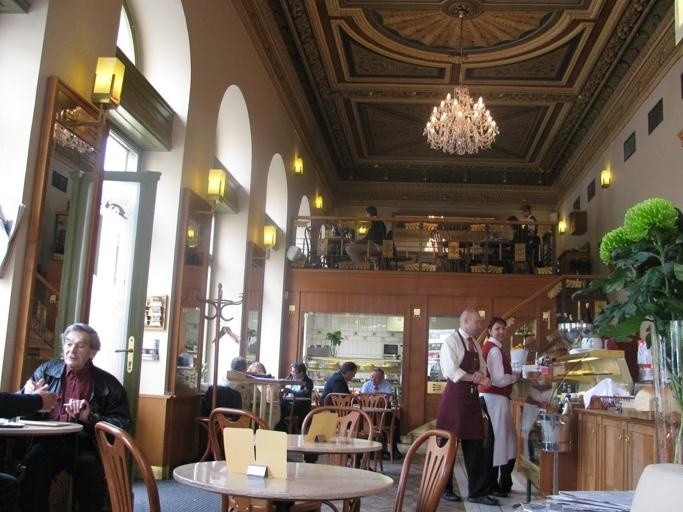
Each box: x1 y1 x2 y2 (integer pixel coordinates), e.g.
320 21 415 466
559 221 566 234
600 170 609 188
65 57 125 127
253 225 277 259
187 220 200 248
315 196 324 209
194 169 226 215
294 157 303 176
423 11 499 156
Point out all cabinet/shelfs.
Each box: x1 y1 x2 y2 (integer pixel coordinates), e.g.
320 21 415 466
527 353 633 408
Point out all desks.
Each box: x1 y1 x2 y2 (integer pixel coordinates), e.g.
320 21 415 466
353 405 393 467
172 461 397 511
0 414 84 510
283 431 384 467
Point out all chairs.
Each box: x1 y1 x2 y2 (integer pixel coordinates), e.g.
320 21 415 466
324 391 398 472
302 405 369 511
303 229 544 273
390 431 459 512
205 406 282 510
94 419 163 512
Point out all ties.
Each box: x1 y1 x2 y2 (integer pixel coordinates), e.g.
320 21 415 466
467 337 475 351
373 384 378 391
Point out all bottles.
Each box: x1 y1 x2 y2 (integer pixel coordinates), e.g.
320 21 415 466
637 338 655 383
556 303 593 333
554 379 575 415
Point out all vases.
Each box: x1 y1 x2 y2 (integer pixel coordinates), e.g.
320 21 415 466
651 320 683 464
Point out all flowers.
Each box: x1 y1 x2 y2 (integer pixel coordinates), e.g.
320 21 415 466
571 197 683 463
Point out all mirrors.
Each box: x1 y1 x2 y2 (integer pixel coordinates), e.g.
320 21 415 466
169 188 212 394
10 75 107 395
242 242 265 367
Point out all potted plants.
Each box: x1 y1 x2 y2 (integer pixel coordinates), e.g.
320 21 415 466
326 331 344 357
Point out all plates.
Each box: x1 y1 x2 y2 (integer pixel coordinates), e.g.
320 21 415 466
0 422 26 429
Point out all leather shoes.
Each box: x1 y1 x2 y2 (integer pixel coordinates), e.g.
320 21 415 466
442 492 459 501
489 488 510 498
467 496 498 505
389 448 402 458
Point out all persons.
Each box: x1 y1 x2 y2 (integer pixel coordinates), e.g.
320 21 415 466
607 329 641 386
520 205 538 268
247 362 274 402
226 355 263 418
0 383 59 512
319 362 372 471
345 205 384 271
497 215 528 272
19 323 130 512
479 316 521 498
361 368 402 461
435 309 499 506
280 362 313 417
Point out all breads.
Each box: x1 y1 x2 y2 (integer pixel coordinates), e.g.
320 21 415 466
635 388 671 411
